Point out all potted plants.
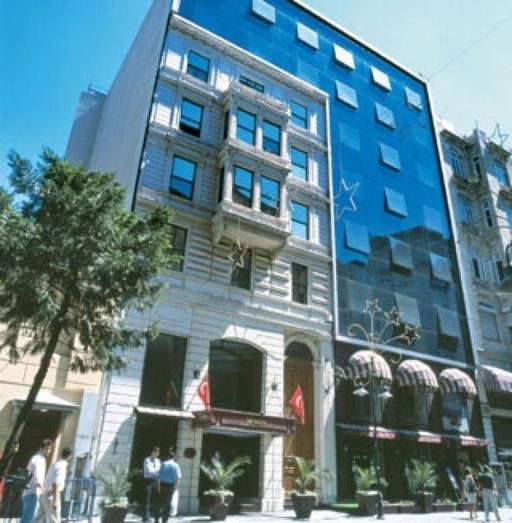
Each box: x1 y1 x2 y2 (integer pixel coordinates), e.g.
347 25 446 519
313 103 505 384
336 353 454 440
405 456 439 513
89 462 144 523
352 458 388 516
283 456 333 518
199 451 252 519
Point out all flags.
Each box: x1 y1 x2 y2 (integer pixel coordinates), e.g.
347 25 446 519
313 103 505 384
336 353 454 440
198 371 211 412
164 380 179 406
288 384 305 426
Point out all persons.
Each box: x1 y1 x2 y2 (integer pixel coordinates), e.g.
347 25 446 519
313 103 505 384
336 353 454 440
19 437 54 522
152 450 182 522
477 463 501 521
143 444 161 522
460 465 479 520
0 439 20 503
37 446 74 522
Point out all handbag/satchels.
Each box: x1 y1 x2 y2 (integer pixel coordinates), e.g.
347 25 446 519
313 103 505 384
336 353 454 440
168 490 179 516
